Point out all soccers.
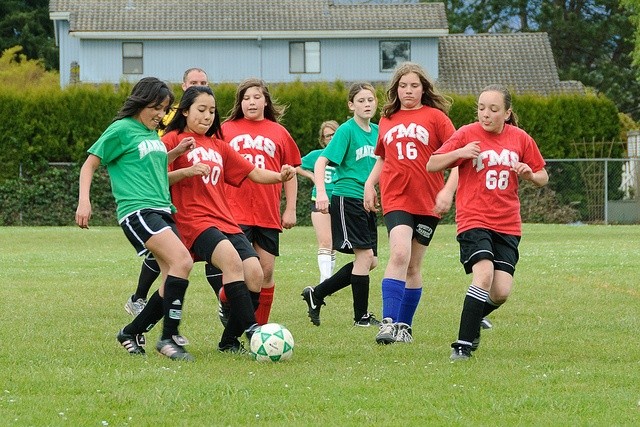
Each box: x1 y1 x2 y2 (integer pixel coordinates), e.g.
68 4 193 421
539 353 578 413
250 323 295 362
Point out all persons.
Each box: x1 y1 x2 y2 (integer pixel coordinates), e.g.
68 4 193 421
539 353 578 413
295 120 340 297
301 82 382 327
426 84 549 364
211 79 302 328
363 62 460 346
160 85 296 357
74 76 198 364
124 68 224 323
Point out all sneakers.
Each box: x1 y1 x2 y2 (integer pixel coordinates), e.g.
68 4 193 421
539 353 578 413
451 339 473 358
376 317 395 343
124 294 149 319
474 320 492 352
218 341 252 361
395 323 414 342
300 286 325 326
154 337 190 362
115 330 147 359
354 314 383 328
219 299 231 328
